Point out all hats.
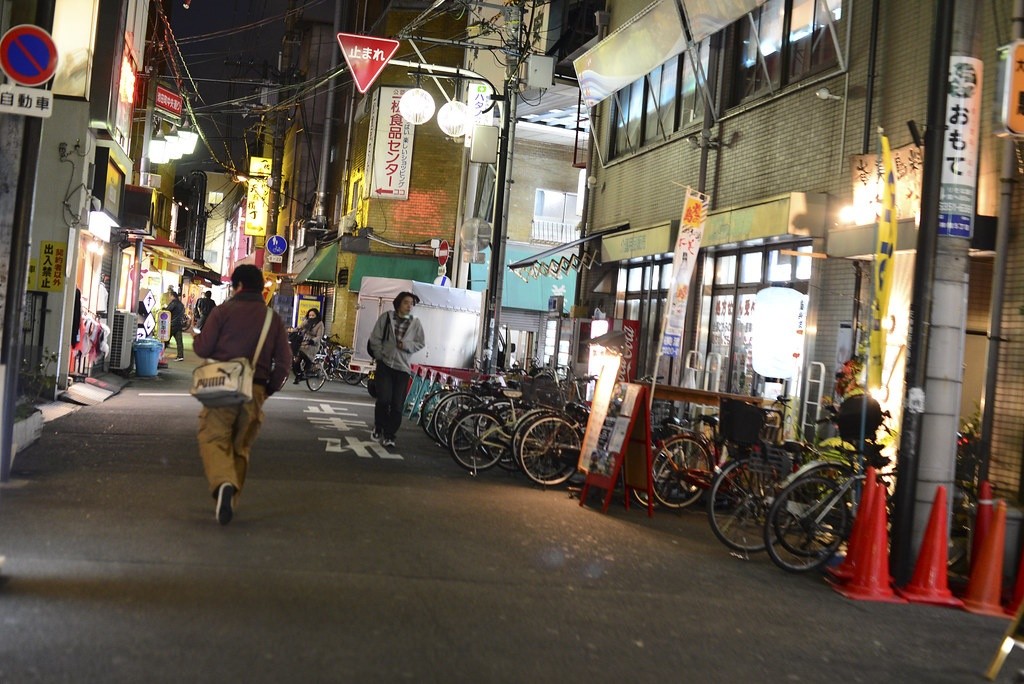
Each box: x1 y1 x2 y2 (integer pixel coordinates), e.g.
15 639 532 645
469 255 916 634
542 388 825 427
393 291 420 313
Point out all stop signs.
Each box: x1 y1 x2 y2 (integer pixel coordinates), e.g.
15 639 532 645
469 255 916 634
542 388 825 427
438 239 449 267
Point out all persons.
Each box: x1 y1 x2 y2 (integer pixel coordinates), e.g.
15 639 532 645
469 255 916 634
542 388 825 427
291 308 324 385
193 265 292 527
160 285 185 362
194 291 216 330
96 274 109 325
369 292 425 449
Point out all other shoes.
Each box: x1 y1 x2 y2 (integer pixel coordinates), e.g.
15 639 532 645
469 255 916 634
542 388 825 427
172 357 184 361
295 373 304 380
216 481 235 527
306 340 314 345
370 426 383 442
382 438 396 447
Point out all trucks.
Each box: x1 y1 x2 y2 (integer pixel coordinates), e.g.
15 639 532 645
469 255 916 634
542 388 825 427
349 275 521 397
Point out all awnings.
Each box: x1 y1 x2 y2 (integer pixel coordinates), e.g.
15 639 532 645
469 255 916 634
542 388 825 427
295 242 339 286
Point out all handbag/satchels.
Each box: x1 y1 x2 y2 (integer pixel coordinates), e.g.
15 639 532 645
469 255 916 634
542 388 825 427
366 310 390 359
197 299 204 318
190 355 254 408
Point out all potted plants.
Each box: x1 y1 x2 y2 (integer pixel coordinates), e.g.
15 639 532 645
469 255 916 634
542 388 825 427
15 347 59 455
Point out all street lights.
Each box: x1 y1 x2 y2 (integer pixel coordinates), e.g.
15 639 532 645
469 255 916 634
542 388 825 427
398 62 512 375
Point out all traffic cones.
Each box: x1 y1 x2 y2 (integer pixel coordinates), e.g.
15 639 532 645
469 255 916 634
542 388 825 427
825 465 1024 617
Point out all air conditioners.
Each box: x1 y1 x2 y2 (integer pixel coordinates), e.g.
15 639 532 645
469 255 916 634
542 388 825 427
109 312 138 370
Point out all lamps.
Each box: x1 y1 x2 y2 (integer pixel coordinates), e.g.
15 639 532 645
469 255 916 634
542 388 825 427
587 177 605 193
119 230 131 250
86 196 103 212
687 130 720 151
148 110 200 166
816 87 843 101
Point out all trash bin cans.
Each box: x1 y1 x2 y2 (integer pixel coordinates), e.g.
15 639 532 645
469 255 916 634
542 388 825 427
132 337 163 377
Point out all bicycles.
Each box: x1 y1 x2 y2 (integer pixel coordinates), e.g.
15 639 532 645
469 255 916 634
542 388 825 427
182 312 191 331
420 355 978 577
280 328 368 392
